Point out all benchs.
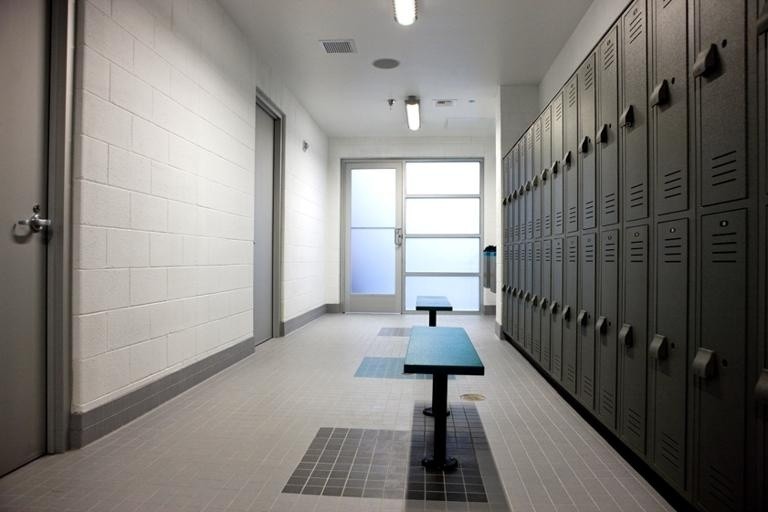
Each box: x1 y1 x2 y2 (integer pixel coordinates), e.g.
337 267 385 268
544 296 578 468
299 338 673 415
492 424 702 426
415 295 453 325
404 325 484 473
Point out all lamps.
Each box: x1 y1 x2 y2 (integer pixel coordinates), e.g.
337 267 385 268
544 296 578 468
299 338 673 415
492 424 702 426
405 96 421 132
393 0 417 26
